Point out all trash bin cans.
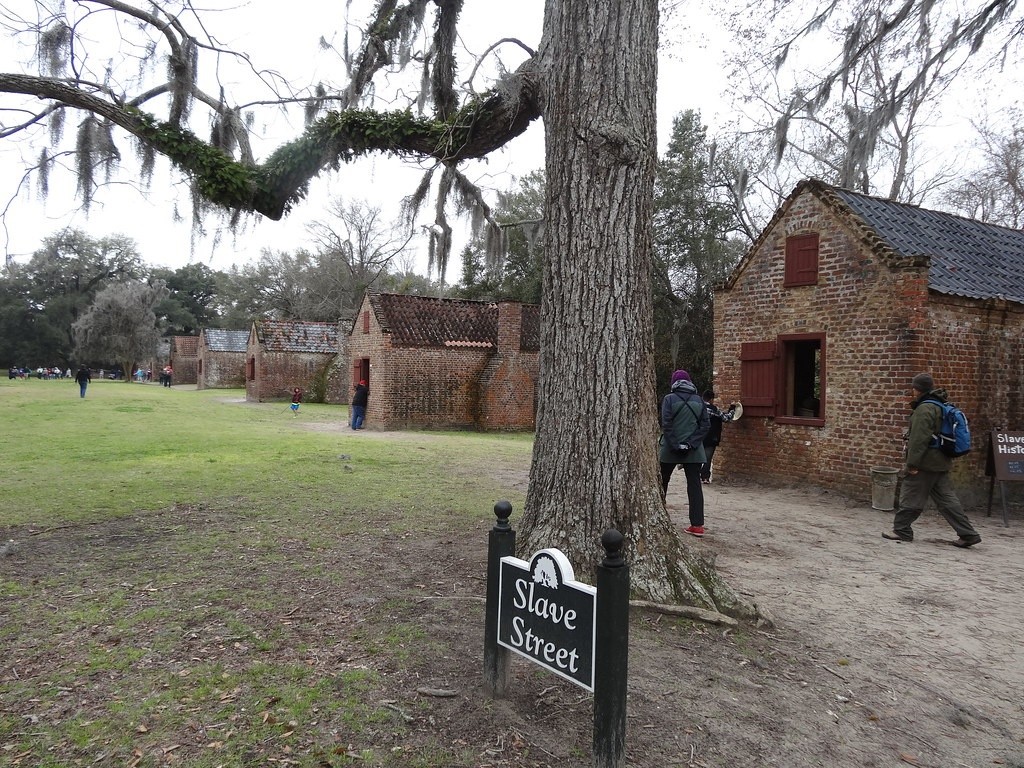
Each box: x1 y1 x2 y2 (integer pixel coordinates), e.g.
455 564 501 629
868 465 899 511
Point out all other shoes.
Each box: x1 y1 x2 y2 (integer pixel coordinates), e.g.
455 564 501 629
702 478 710 484
684 525 705 537
356 427 365 431
882 529 913 541
953 535 982 547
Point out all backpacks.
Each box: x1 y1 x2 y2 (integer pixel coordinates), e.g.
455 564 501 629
919 398 972 457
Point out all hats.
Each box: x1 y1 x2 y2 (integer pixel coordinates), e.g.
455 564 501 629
912 372 934 391
360 380 367 385
80 364 87 369
671 370 692 382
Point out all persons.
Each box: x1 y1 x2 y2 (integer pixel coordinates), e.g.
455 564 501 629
657 370 736 536
290 387 302 419
159 365 173 388
352 380 368 431
133 369 152 383
8 365 115 380
882 373 982 547
75 364 91 398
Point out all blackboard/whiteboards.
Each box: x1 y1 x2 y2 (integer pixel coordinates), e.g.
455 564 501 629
990 430 1024 480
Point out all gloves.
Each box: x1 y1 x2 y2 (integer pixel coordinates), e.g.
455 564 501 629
676 442 691 457
